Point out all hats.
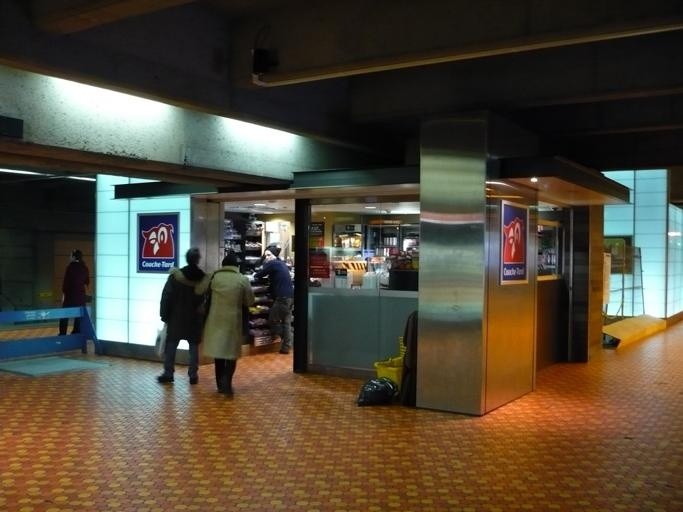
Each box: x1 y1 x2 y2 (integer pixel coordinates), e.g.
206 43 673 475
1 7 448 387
222 253 238 267
268 247 281 257
187 247 199 263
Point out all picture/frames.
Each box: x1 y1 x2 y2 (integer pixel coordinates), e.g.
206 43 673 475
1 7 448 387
499 200 529 286
136 210 179 272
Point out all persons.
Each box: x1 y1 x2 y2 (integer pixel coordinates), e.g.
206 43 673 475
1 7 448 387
253 247 294 354
167 255 255 394
154 247 210 384
58 249 90 336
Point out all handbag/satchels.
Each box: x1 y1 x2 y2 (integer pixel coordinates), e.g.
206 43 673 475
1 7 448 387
196 289 212 313
84 295 92 302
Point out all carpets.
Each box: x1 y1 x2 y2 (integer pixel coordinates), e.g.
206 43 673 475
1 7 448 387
0 355 113 377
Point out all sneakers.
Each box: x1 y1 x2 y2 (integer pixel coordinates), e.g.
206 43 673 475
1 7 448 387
158 375 174 382
190 375 198 383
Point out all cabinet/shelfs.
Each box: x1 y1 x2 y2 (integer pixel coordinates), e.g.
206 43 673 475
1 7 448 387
309 225 419 290
221 214 294 357
